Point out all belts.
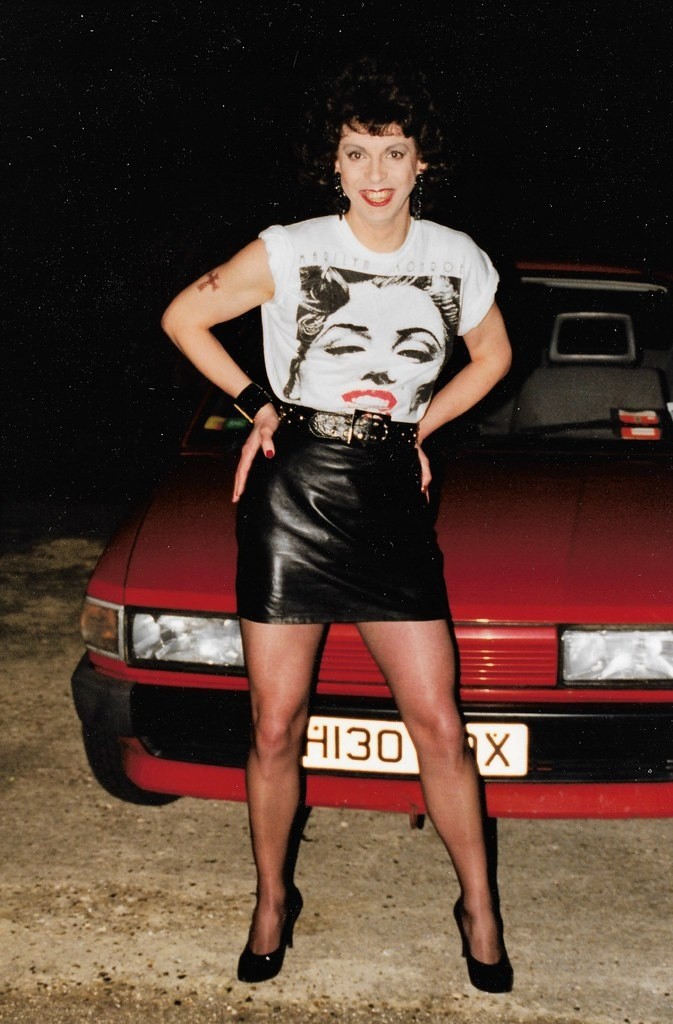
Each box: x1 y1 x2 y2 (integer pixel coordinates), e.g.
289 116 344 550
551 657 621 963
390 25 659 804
276 398 419 448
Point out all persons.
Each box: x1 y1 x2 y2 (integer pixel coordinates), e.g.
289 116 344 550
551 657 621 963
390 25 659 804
164 61 514 993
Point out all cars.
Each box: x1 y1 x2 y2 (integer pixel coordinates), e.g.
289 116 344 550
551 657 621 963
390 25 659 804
69 254 673 818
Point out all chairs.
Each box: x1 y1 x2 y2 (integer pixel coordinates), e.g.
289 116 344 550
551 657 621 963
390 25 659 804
513 312 673 428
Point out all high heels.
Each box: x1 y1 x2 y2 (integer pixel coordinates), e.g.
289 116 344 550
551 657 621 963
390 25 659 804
452 898 514 994
236 880 304 984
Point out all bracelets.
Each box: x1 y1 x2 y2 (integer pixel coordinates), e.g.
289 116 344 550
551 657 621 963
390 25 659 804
234 382 272 424
